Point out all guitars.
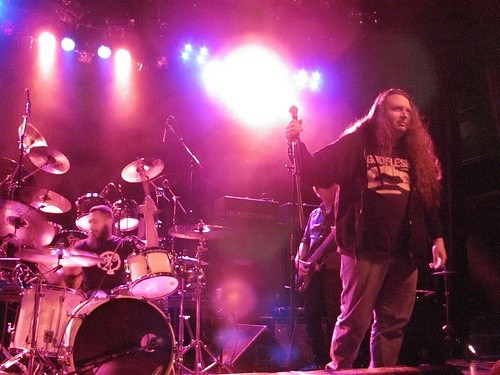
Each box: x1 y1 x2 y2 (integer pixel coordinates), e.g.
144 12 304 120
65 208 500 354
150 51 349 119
295 226 336 291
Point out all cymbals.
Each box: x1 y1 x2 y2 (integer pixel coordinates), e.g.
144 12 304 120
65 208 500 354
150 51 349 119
121 157 165 182
15 248 105 264
20 187 71 214
177 256 207 266
168 224 234 240
18 123 70 175
0 198 55 246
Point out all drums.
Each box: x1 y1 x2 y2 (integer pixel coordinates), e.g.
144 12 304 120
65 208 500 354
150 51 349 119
125 245 181 299
59 295 176 375
9 284 88 357
174 269 202 296
114 198 141 233
76 193 110 231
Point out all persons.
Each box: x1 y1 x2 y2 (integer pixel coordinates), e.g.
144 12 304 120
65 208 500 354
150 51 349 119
294 186 343 370
38 197 159 297
285 89 447 369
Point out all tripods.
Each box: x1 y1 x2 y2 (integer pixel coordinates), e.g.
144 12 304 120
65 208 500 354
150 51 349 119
1 239 232 374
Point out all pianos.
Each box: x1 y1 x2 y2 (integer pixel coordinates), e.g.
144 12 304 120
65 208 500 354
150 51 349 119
210 195 320 316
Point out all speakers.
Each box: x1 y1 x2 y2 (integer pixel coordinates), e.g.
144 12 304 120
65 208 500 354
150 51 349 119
223 323 289 373
259 316 328 367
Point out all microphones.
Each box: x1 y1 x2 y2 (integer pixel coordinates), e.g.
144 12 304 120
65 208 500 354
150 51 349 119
25 88 31 118
289 105 298 140
